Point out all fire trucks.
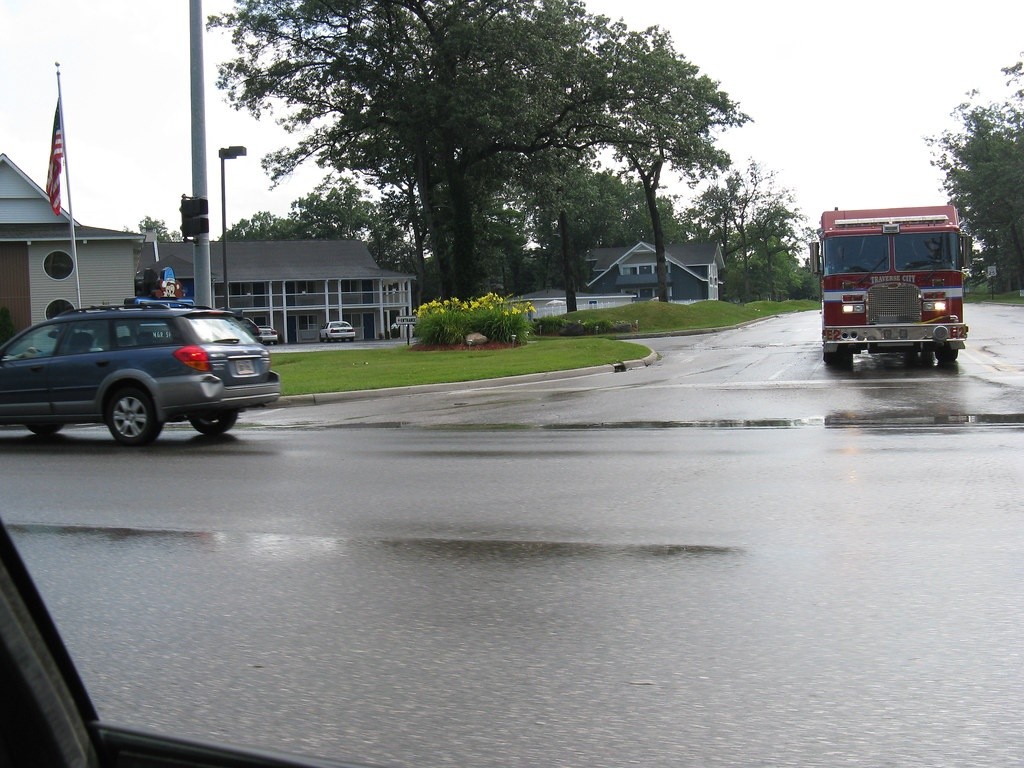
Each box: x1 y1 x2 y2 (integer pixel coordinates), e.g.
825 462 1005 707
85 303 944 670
808 206 973 366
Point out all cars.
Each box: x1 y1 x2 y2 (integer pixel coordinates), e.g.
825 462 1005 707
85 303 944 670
258 326 279 345
319 321 356 343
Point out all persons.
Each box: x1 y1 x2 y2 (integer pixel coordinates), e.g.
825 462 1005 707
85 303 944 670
832 244 873 273
921 241 949 268
16 327 74 359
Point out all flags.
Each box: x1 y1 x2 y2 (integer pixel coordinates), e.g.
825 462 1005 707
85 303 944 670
46 97 65 216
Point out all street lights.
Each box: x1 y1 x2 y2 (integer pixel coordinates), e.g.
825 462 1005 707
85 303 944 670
218 146 248 311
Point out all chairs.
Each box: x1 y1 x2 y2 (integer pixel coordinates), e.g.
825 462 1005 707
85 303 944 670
136 331 153 346
66 332 94 355
119 336 133 346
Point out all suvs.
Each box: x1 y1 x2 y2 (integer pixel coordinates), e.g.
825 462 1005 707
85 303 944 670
243 318 264 343
0 267 283 448
